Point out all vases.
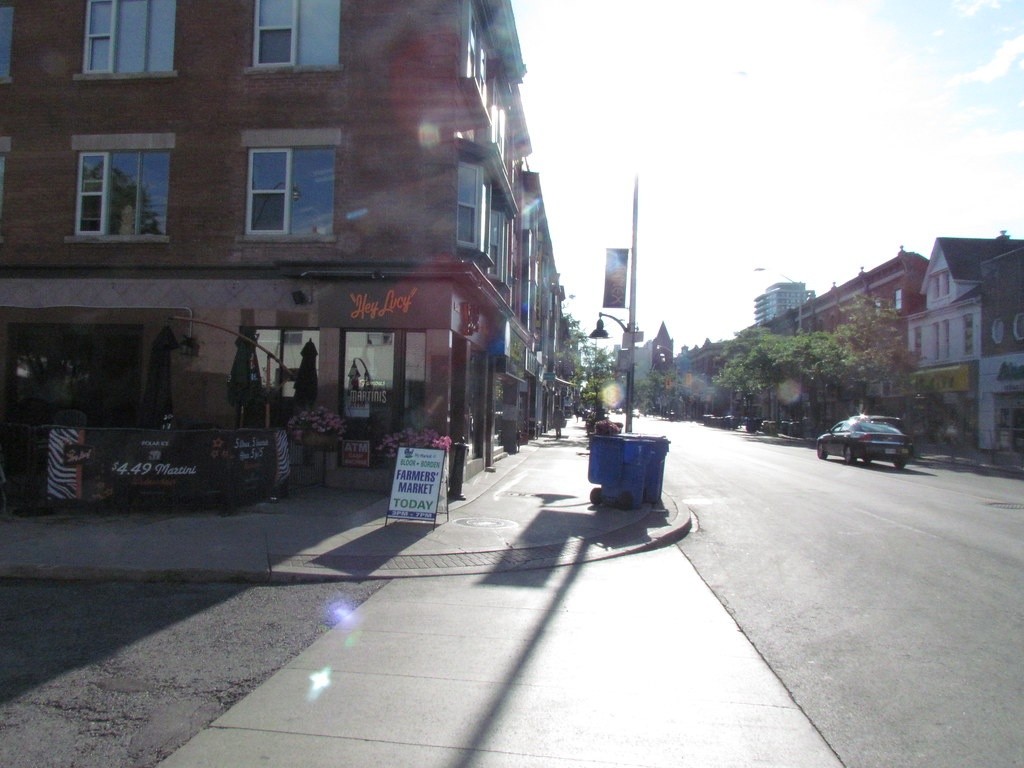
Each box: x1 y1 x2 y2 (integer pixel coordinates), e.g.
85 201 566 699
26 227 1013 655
303 431 339 448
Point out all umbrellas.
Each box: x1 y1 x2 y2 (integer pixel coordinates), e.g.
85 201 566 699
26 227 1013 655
347 359 361 399
226 327 264 427
141 324 182 429
293 342 319 409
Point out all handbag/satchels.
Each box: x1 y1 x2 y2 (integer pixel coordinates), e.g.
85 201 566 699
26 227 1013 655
558 417 567 428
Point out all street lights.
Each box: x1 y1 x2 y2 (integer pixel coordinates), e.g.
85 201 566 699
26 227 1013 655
587 176 645 432
754 268 802 437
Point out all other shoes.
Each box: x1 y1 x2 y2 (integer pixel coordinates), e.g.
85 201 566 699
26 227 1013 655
556 435 561 439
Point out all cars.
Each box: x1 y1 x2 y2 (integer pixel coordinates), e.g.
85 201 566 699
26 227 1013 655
817 420 914 470
849 415 908 435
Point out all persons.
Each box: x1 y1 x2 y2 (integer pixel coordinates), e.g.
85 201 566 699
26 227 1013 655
552 404 567 442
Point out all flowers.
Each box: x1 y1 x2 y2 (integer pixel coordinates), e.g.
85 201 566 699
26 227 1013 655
374 427 452 457
287 405 346 442
594 417 621 436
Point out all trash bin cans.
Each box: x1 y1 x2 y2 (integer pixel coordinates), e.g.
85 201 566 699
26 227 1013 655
588 433 671 509
702 414 800 436
